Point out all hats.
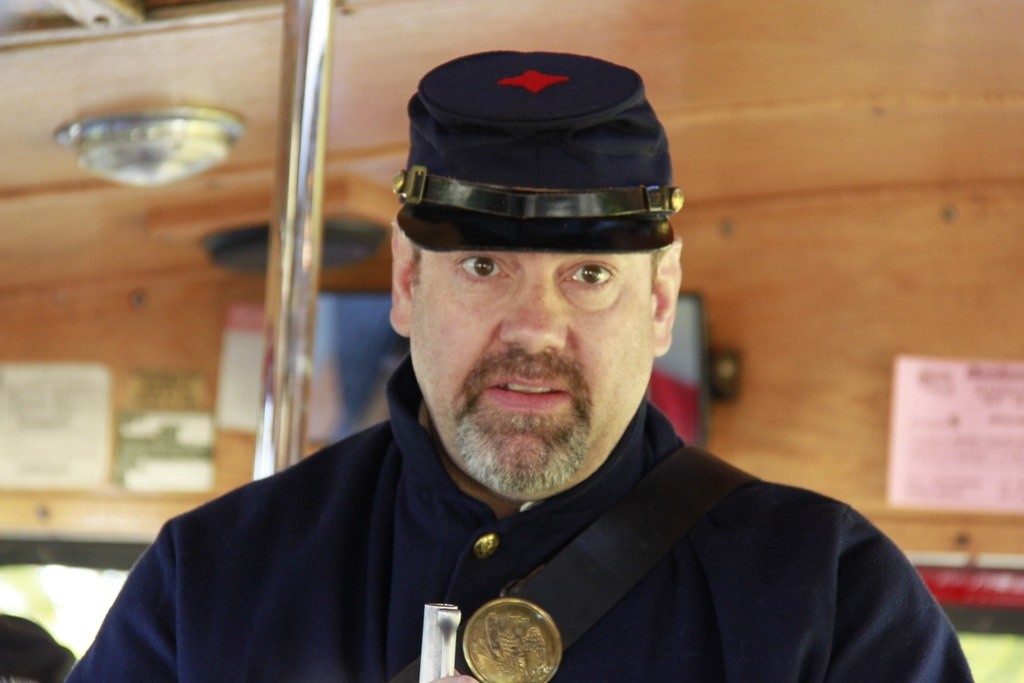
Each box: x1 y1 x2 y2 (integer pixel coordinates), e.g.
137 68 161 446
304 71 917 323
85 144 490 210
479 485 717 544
393 47 681 255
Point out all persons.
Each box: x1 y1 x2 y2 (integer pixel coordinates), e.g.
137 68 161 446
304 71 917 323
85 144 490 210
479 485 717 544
65 50 975 683
